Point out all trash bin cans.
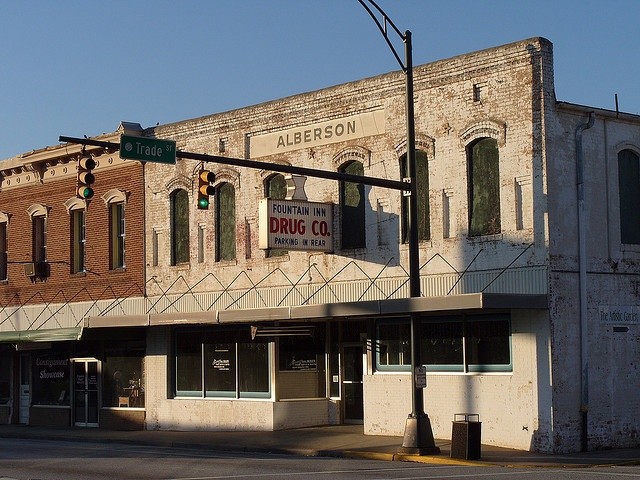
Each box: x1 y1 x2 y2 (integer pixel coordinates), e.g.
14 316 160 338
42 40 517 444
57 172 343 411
450 413 481 460
